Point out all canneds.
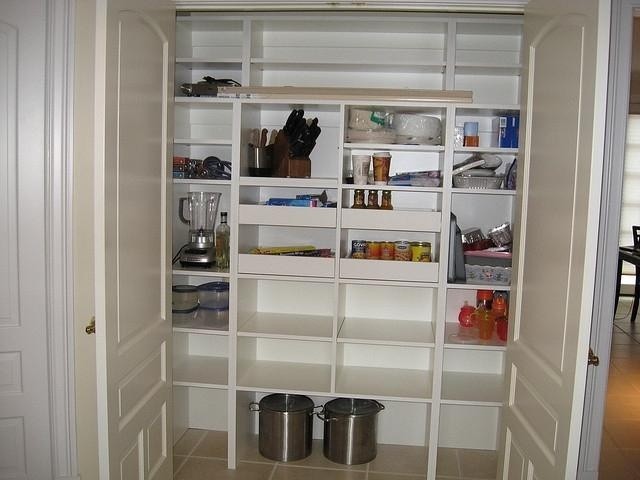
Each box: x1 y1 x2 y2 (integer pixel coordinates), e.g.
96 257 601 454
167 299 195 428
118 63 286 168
351 189 366 209
380 242 395 260
352 240 366 258
411 242 431 261
395 241 410 260
366 190 380 208
379 190 393 209
366 241 380 259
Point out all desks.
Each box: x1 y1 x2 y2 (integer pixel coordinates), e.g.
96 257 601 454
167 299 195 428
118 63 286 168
614 246 640 322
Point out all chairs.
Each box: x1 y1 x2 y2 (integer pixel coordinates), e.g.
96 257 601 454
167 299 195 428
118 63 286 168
631 225 640 323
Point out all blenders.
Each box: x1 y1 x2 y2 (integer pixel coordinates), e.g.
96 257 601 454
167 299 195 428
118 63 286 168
177 191 221 266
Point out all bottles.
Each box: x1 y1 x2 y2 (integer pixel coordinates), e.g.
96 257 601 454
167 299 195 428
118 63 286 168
354 189 365 205
216 212 229 269
368 190 378 207
457 305 476 327
381 191 392 206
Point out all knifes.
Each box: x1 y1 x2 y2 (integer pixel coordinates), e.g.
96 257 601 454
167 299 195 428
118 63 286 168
282 107 322 159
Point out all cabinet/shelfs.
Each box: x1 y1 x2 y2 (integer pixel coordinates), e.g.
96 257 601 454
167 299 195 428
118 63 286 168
172 16 524 480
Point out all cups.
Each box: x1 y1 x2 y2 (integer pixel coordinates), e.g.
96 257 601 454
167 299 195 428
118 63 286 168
372 151 393 185
248 146 274 176
351 154 371 184
476 289 510 343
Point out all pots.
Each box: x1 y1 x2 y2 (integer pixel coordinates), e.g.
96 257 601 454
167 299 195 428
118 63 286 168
248 393 326 462
316 398 386 466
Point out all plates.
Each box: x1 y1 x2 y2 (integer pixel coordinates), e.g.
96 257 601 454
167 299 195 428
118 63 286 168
446 332 478 343
